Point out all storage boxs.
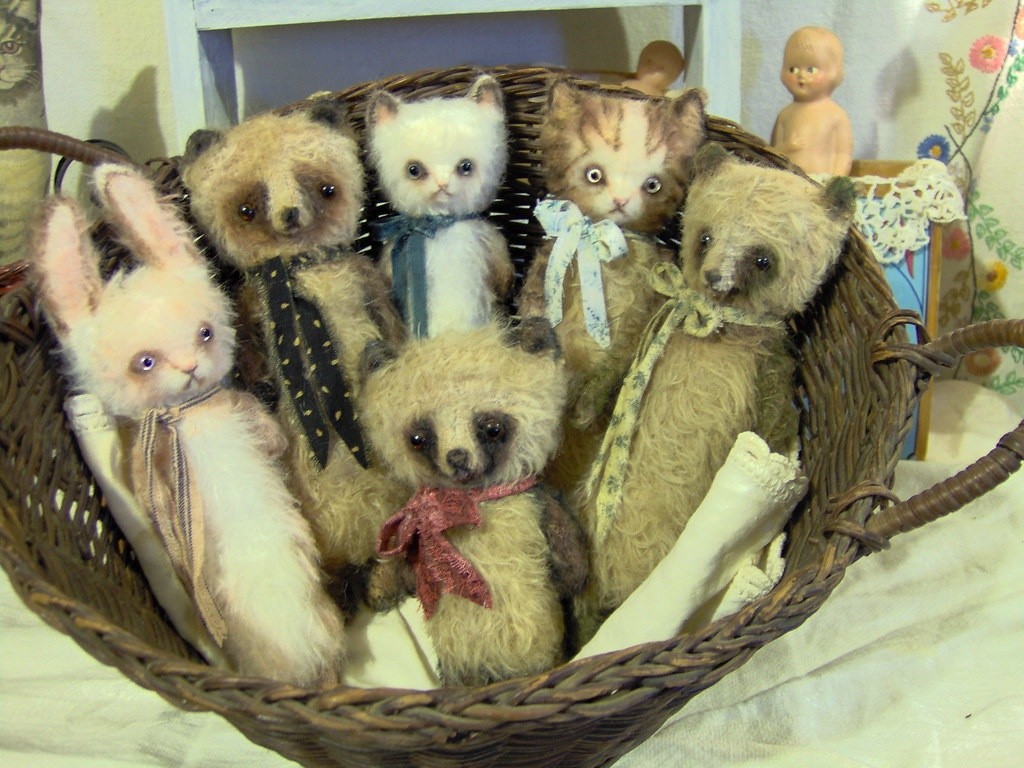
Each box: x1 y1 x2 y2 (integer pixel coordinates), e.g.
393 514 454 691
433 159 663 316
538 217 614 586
842 159 943 463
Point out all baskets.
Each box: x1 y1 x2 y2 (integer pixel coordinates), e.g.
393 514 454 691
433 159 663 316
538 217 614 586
0 64 1024 768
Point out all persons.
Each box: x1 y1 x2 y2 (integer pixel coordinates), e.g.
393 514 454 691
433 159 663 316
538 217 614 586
619 41 683 100
771 26 853 179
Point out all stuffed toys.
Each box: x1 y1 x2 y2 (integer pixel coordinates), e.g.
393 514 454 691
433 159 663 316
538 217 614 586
356 316 589 690
522 76 704 508
365 72 513 337
181 90 417 595
36 162 345 686
569 141 854 619
